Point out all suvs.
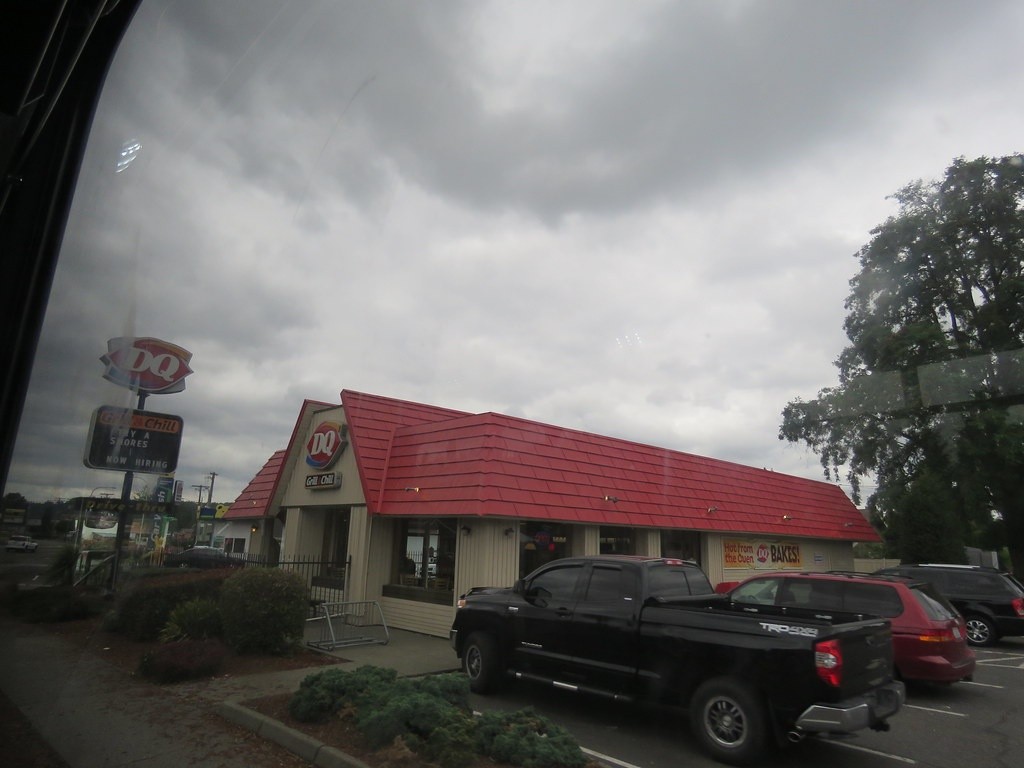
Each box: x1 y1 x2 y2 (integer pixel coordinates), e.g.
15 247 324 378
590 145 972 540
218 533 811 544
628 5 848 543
866 561 1024 646
724 569 976 686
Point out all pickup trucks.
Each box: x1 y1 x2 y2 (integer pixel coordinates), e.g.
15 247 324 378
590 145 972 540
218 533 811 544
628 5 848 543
449 553 909 767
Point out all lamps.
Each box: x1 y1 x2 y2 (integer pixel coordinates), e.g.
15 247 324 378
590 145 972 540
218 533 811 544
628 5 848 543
250 523 259 533
783 515 791 520
708 506 718 513
461 524 472 536
845 522 854 527
505 527 514 538
604 496 618 504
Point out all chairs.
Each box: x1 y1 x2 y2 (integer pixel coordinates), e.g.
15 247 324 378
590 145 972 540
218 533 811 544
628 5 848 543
781 592 798 608
808 588 833 609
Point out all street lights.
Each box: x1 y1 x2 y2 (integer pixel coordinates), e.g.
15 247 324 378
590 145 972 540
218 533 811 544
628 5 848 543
71 486 116 560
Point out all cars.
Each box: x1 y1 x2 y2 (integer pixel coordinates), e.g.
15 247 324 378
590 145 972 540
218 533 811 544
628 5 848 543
6 535 38 553
64 516 136 558
164 547 245 570
